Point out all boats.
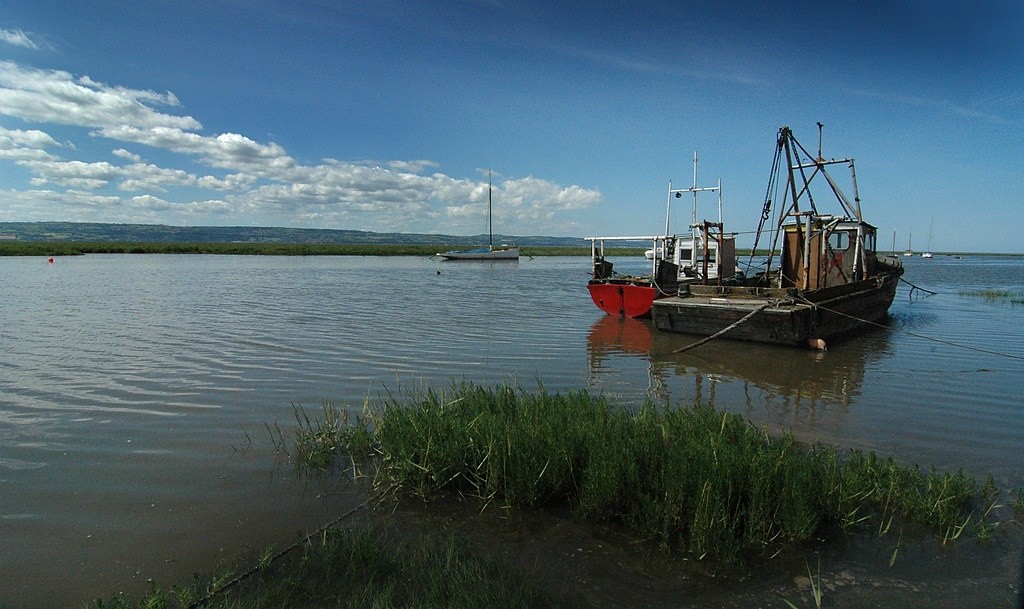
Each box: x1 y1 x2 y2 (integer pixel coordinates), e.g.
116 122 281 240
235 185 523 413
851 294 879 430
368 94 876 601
644 247 668 260
582 149 744 318
649 121 904 354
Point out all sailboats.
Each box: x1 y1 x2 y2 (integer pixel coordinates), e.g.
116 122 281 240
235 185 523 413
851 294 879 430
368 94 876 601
921 216 934 258
885 230 900 258
436 168 522 260
903 232 914 257
